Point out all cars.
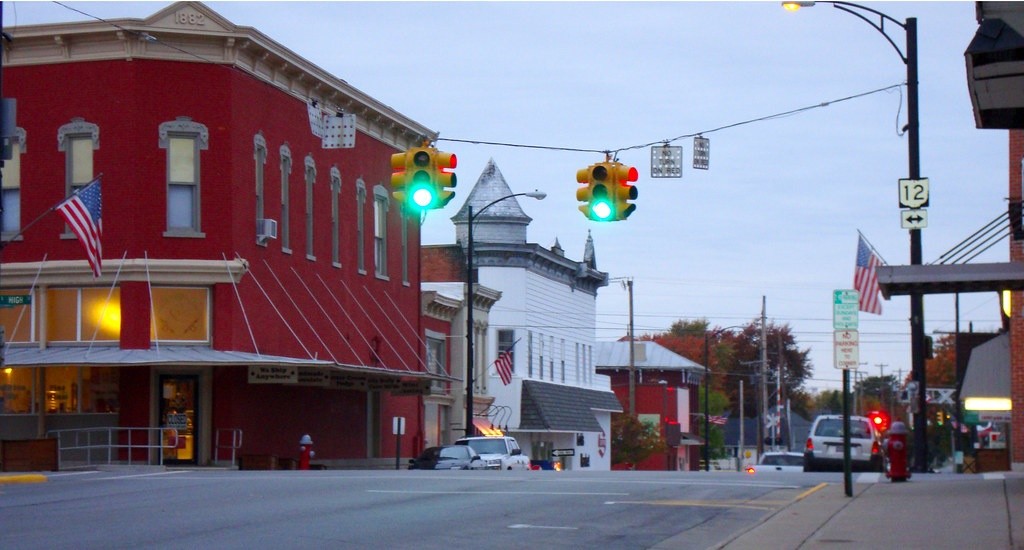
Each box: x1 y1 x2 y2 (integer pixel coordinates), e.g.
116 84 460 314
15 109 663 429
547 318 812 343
408 445 489 470
757 453 805 474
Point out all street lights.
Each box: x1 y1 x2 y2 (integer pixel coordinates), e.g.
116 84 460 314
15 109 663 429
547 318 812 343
704 325 753 473
467 186 547 430
782 1 932 474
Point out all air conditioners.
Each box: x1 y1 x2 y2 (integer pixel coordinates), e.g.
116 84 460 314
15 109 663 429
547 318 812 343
257 219 277 240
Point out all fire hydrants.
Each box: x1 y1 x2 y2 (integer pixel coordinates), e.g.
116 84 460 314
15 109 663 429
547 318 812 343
886 418 912 483
296 435 316 470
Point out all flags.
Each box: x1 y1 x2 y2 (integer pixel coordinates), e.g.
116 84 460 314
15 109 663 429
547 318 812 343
494 347 512 386
708 412 729 425
55 179 102 278
854 235 883 315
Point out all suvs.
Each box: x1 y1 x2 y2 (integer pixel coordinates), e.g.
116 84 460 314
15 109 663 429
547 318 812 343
802 414 885 472
455 437 532 471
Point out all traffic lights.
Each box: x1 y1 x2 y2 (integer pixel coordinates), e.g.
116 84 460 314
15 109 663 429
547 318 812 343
946 414 951 419
436 152 458 208
391 153 406 203
575 166 589 220
872 413 886 430
898 390 911 403
617 165 639 222
591 165 614 220
936 412 943 426
411 150 435 208
